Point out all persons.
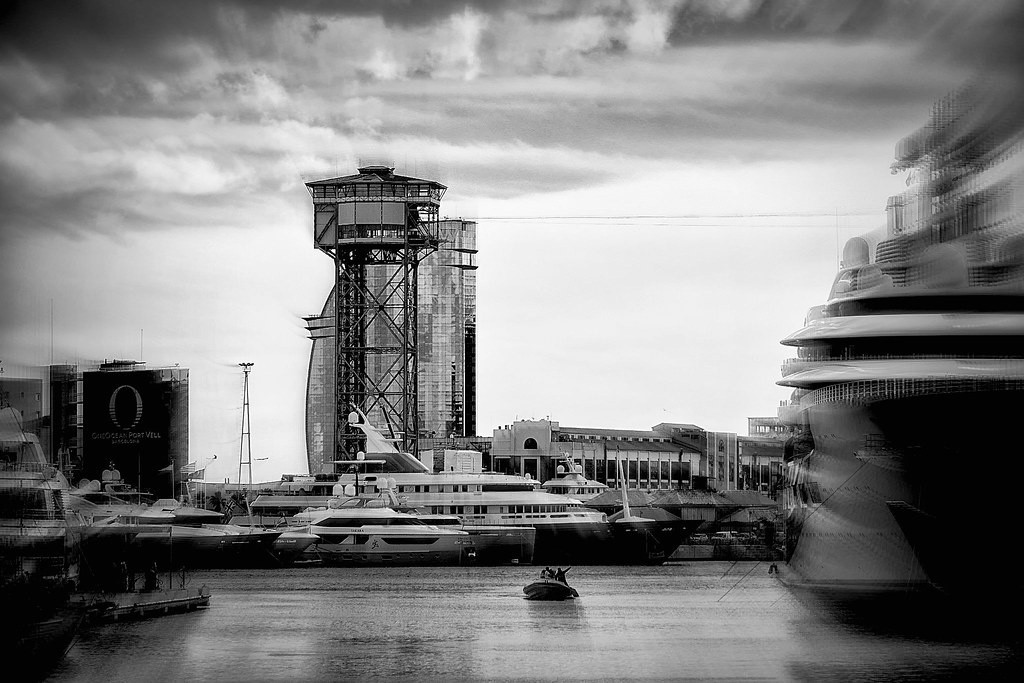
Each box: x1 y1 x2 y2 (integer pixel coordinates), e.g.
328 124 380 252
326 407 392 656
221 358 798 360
545 566 556 580
143 560 159 594
325 500 329 510
555 565 572 587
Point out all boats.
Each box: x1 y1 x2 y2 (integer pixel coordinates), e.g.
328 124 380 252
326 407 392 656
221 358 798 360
0 407 709 580
777 88 1023 625
1 600 91 673
523 580 576 600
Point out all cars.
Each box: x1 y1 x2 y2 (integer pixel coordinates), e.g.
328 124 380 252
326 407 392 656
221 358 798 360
691 530 761 545
